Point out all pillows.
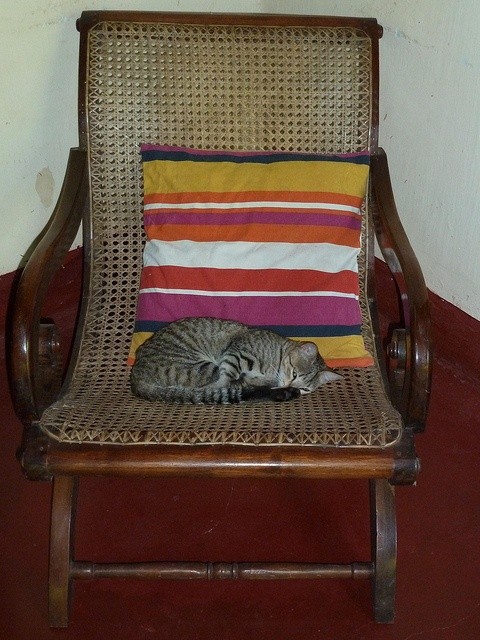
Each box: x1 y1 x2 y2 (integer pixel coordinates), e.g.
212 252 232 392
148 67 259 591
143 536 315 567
126 143 376 371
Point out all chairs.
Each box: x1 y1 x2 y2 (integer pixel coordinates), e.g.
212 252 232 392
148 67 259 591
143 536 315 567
1 9 433 630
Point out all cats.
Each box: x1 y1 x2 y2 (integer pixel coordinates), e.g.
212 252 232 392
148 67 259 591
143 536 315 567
130 316 346 406
